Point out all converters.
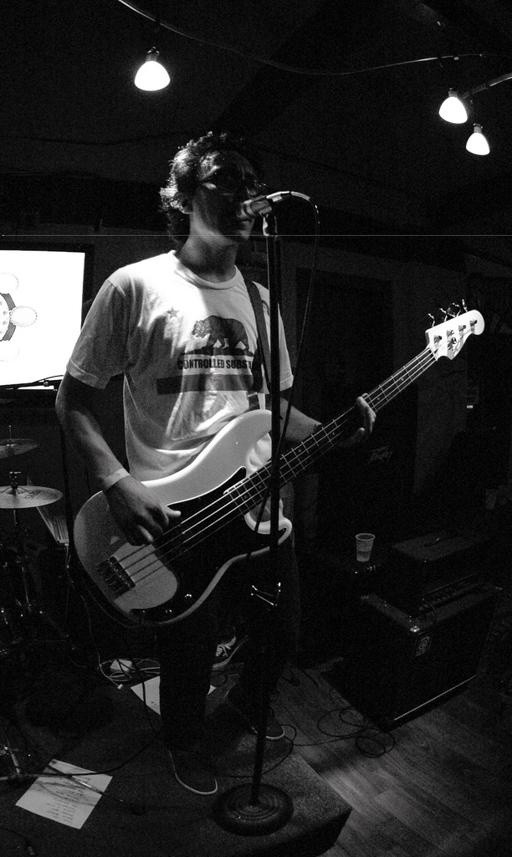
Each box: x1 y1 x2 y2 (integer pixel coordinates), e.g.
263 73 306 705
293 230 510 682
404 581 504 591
111 659 134 673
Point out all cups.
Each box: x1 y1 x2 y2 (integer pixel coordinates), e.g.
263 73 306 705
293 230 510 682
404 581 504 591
354 532 376 563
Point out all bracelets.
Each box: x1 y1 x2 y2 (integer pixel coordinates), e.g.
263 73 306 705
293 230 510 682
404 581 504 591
100 468 129 493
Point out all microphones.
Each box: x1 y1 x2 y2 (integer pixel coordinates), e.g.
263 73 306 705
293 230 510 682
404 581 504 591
234 188 293 223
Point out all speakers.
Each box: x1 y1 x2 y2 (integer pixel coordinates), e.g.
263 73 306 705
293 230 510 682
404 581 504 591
339 590 501 732
387 528 486 611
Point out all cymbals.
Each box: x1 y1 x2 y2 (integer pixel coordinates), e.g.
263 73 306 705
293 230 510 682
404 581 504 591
1 438 38 458
1 485 63 509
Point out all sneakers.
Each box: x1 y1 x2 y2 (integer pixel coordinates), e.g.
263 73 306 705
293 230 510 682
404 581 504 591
212 636 247 669
166 745 218 795
224 689 285 740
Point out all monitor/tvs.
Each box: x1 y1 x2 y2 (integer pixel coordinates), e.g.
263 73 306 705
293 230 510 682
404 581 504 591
1 243 98 393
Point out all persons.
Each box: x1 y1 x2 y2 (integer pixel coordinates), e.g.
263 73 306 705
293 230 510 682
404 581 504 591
54 126 378 796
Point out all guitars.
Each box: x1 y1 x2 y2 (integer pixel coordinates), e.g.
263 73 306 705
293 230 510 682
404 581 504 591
73 299 486 625
